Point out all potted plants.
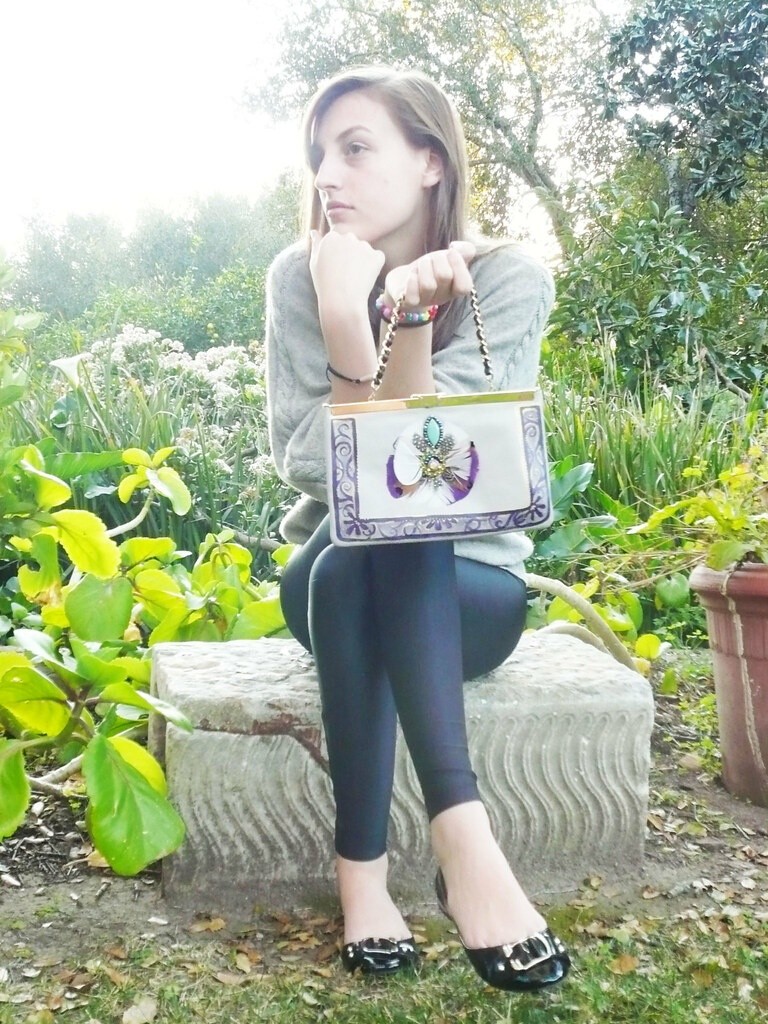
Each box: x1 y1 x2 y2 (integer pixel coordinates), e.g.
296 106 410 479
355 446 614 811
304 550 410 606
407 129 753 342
635 408 767 808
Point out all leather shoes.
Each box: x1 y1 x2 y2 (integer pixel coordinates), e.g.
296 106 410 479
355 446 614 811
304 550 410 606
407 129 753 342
341 935 423 984
435 865 571 994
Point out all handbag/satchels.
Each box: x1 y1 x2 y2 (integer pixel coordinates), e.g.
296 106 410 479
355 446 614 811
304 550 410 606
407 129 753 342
322 285 554 548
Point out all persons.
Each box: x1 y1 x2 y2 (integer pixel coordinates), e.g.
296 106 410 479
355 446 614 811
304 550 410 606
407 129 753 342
266 65 602 996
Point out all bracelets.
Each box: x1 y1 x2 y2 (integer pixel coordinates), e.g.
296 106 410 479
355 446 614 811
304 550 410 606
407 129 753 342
322 359 383 387
373 294 438 329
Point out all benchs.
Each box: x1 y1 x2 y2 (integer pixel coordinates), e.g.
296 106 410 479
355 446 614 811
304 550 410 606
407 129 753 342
146 628 656 902
